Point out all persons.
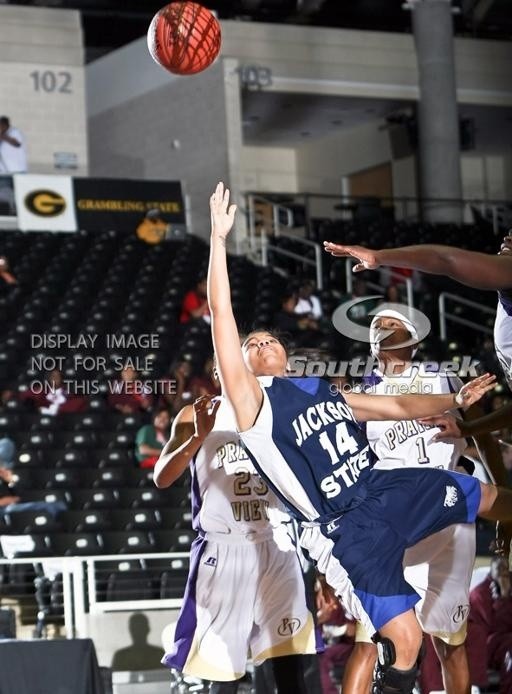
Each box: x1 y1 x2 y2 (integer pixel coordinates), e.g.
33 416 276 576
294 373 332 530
180 276 212 325
107 364 153 415
199 358 221 398
0 115 31 218
159 359 204 413
206 181 512 694
339 276 378 322
148 324 327 694
0 466 31 514
23 369 85 416
284 278 326 333
133 408 171 467
385 280 403 304
321 224 512 400
341 308 512 694
305 535 512 694
135 206 168 246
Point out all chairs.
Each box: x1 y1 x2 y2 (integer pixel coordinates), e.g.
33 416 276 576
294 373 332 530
1 219 511 638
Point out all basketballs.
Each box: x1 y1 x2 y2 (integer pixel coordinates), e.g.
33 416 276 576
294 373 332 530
146 0 221 76
